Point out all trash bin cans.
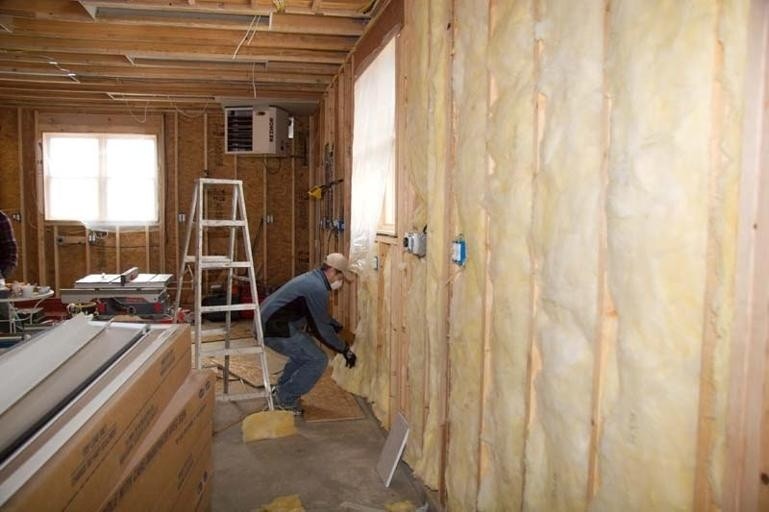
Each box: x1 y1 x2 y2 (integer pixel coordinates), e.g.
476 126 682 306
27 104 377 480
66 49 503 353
69 302 96 318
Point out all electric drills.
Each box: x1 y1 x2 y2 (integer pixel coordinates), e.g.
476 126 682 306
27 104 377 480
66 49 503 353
304 179 344 202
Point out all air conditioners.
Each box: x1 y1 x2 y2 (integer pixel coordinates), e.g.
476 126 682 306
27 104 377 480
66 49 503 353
222 104 288 160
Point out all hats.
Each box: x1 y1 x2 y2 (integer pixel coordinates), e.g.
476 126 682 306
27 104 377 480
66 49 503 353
323 252 353 281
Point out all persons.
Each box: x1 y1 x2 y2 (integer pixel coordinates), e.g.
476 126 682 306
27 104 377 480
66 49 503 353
250 252 358 418
1 209 23 335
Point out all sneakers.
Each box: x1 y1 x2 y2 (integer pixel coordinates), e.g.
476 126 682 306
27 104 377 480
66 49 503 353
265 386 304 416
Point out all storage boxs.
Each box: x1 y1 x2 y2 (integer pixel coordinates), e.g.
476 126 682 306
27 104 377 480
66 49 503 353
98 367 218 512
2 319 192 511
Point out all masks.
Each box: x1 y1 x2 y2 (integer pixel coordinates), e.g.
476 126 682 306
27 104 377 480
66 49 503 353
330 279 343 291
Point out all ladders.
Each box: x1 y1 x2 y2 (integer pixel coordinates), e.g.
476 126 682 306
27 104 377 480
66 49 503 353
173 176 275 413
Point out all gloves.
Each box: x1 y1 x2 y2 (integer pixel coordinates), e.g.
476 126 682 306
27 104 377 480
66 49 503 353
343 345 357 368
